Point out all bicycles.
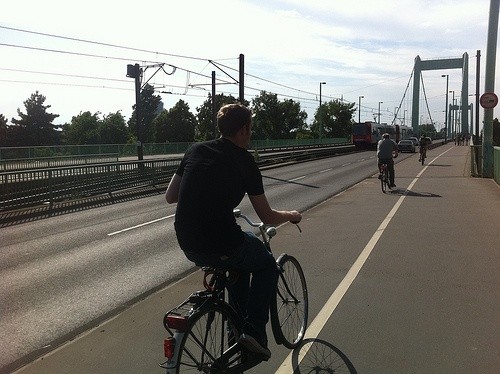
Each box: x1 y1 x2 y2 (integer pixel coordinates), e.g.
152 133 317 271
378 155 397 193
419 146 427 165
158 207 308 374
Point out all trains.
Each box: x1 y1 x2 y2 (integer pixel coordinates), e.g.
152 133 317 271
351 121 414 147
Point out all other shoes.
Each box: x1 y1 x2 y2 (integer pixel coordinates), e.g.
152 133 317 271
390 182 396 187
377 173 383 180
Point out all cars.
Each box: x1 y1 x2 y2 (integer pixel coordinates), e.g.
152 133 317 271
398 140 416 153
407 137 419 145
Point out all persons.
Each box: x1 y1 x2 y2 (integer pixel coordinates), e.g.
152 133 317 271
454 132 470 146
165 104 301 357
417 135 429 162
377 133 398 188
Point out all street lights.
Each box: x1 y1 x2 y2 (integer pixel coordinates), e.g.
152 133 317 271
442 74 449 144
318 81 327 143
394 107 398 124
450 90 458 139
358 96 365 123
378 102 383 124
403 109 408 125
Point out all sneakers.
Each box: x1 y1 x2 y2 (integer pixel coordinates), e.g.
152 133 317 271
233 325 271 358
